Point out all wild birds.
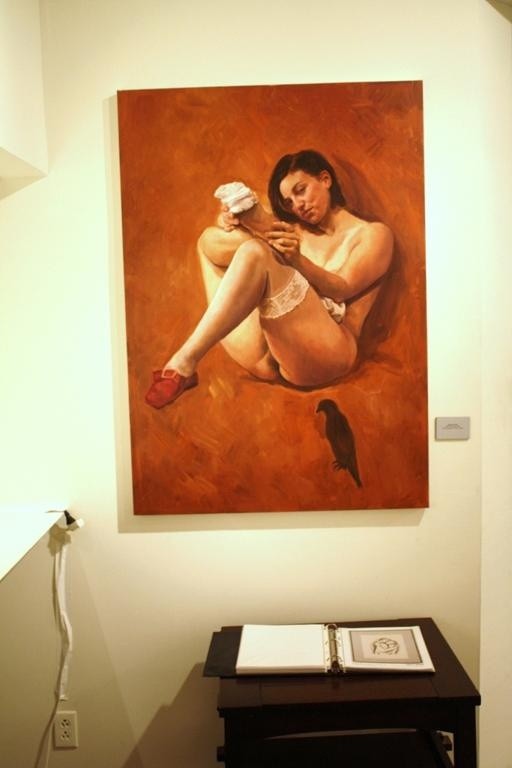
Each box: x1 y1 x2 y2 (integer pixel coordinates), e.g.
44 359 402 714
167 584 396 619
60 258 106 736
314 398 363 489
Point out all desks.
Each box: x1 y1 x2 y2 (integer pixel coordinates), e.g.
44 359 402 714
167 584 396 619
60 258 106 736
216 616 482 767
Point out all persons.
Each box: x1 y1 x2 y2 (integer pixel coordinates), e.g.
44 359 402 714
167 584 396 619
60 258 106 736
144 148 395 410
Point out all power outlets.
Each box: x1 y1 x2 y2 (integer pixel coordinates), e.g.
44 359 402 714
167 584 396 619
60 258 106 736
53 710 79 749
